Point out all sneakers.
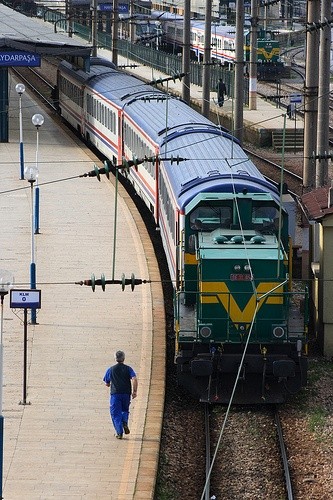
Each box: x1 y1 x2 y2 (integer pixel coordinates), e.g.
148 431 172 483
125 429 130 434
115 433 121 439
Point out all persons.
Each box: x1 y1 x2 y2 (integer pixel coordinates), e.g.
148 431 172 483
102 349 139 441
215 78 227 108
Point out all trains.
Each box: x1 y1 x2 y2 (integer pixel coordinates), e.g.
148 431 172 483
150 9 286 77
51 56 318 407
110 11 162 52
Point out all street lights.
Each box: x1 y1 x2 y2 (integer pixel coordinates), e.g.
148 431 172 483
14 83 28 181
0 270 15 500
31 112 45 234
24 165 42 325
